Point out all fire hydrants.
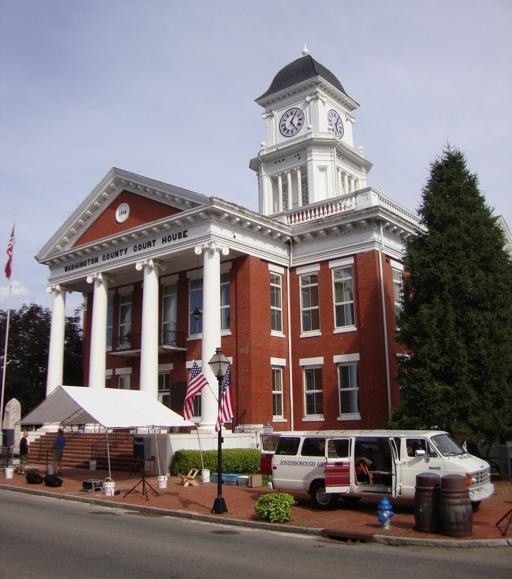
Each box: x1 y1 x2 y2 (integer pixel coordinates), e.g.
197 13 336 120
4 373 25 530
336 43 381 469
376 497 394 529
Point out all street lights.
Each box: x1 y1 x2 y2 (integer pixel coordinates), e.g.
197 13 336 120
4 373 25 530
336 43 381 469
206 347 231 514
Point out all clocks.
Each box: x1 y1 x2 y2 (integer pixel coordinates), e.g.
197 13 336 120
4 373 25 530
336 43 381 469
277 105 305 137
326 108 345 141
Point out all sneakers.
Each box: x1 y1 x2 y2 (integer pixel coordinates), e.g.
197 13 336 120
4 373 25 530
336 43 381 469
17 472 63 475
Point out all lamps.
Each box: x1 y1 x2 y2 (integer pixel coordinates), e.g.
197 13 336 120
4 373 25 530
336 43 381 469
191 255 203 323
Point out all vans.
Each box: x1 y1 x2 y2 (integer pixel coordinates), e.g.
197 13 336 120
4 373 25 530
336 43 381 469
260 427 496 512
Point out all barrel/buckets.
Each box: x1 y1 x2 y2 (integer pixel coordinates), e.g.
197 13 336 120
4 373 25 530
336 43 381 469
5 468 13 479
89 460 97 470
103 477 115 496
201 469 210 482
237 476 249 487
415 485 439 533
158 476 168 488
47 464 54 475
440 489 475 537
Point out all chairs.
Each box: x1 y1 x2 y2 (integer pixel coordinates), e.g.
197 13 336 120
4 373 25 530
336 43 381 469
361 461 392 486
177 468 201 489
354 446 367 476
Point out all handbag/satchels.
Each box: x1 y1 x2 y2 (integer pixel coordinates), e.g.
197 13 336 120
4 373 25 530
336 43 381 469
45 474 64 487
26 472 44 484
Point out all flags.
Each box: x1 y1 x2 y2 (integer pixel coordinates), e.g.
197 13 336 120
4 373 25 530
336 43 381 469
183 361 208 420
214 366 234 432
4 225 14 278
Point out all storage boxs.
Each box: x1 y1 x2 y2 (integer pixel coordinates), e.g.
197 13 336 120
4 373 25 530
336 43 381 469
83 478 103 489
238 475 248 486
222 473 240 487
249 474 263 490
212 472 218 482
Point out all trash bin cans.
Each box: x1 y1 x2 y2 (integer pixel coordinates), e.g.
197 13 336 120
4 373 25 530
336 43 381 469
438 473 473 538
413 472 440 532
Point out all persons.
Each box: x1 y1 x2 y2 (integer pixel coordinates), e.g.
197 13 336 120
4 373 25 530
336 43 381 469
410 443 420 456
53 428 65 475
18 432 28 475
